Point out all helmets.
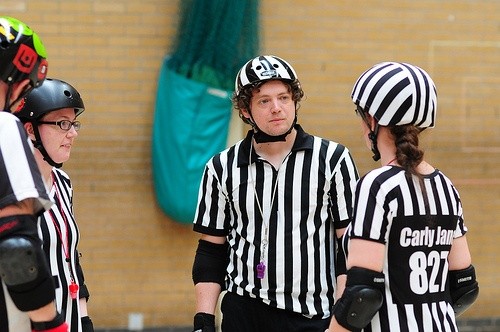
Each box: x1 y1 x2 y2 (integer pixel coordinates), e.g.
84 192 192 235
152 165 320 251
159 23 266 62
233 55 301 94
0 15 48 88
14 78 85 121
351 61 437 130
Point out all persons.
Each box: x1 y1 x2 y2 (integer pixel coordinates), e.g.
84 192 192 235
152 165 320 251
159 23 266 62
0 16 68 332
329 61 479 332
14 79 94 332
192 54 360 332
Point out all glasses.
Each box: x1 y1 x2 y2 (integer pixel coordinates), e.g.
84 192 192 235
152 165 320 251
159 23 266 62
40 119 81 132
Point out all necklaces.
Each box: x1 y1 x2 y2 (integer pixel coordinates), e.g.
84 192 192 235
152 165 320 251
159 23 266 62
249 136 283 278
388 158 396 164
